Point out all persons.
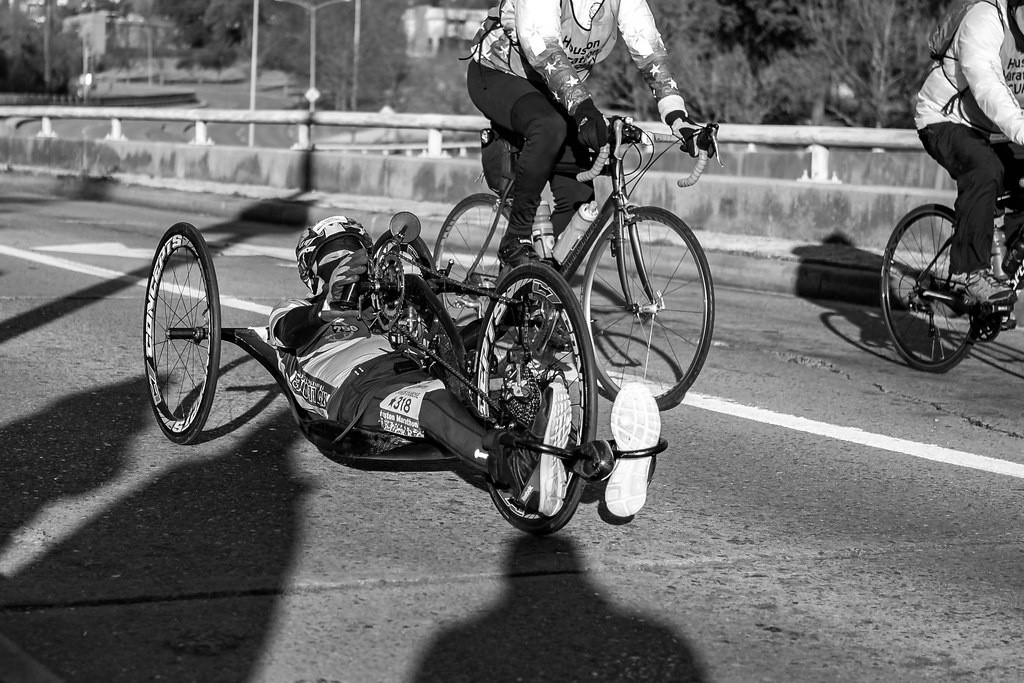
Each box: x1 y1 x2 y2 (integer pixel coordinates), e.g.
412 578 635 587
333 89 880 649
916 0 1024 329
467 0 717 270
269 215 661 517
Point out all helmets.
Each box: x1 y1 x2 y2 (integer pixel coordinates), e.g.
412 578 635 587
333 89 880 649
295 215 376 296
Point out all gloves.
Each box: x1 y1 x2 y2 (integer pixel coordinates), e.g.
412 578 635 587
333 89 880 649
665 110 717 159
574 97 611 155
327 248 369 307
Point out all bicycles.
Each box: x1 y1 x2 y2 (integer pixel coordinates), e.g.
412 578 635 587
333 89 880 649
142 206 670 540
434 114 718 414
878 130 1024 376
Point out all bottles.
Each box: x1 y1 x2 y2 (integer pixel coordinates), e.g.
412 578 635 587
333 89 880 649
991 225 1008 278
1002 225 1024 276
531 201 555 259
552 200 600 263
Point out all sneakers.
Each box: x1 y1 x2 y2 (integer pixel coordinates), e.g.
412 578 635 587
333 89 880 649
945 267 1018 306
506 381 573 519
497 234 541 266
605 381 661 518
